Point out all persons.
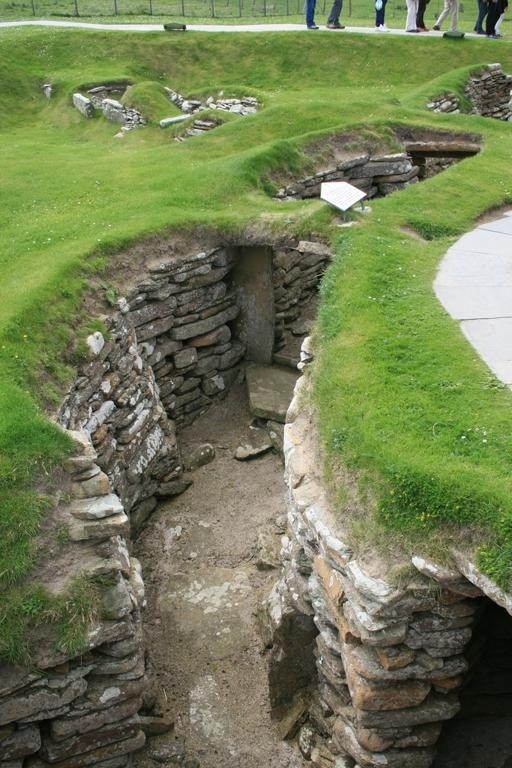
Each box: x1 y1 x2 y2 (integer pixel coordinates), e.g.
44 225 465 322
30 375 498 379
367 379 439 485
404 0 423 33
488 0 508 38
416 0 430 31
372 0 391 32
473 0 489 34
326 0 346 30
433 0 458 31
303 0 319 29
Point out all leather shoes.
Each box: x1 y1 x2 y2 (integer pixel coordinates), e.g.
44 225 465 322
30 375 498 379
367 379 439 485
326 22 346 29
307 25 319 30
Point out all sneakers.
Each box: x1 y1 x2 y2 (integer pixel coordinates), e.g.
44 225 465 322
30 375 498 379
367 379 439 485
373 22 392 33
405 24 440 33
472 25 503 39
